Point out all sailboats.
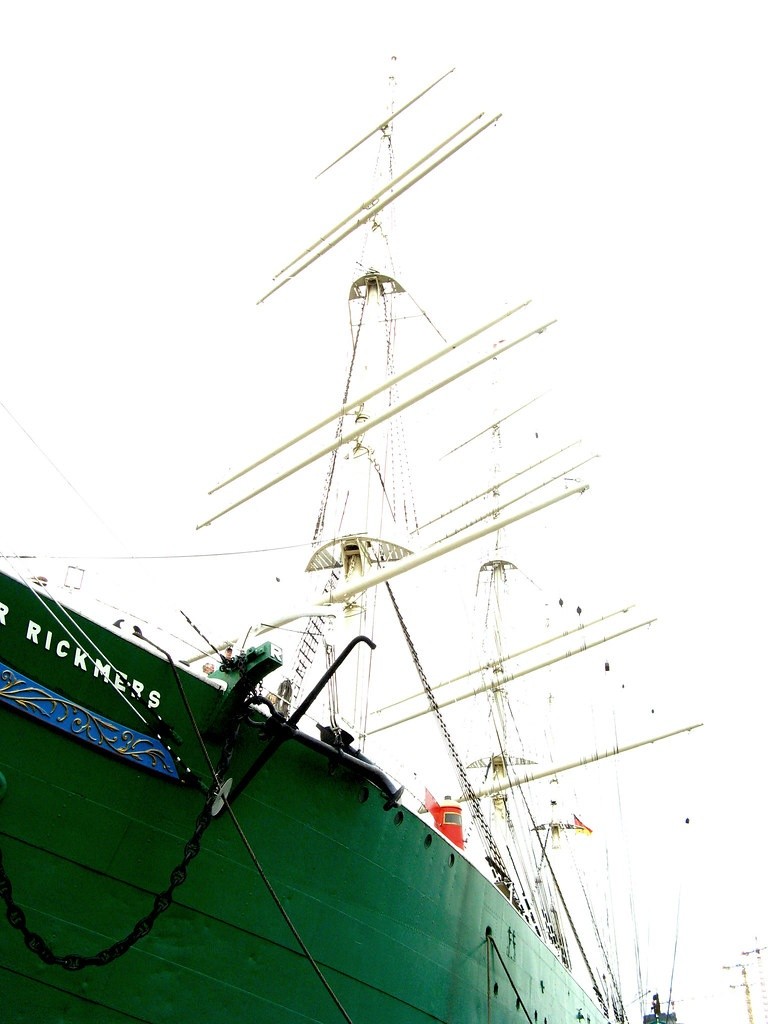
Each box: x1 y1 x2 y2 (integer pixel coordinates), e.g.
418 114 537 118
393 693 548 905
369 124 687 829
0 50 717 1024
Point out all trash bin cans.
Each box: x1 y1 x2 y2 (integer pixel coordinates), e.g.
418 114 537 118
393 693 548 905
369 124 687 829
434 800 464 851
